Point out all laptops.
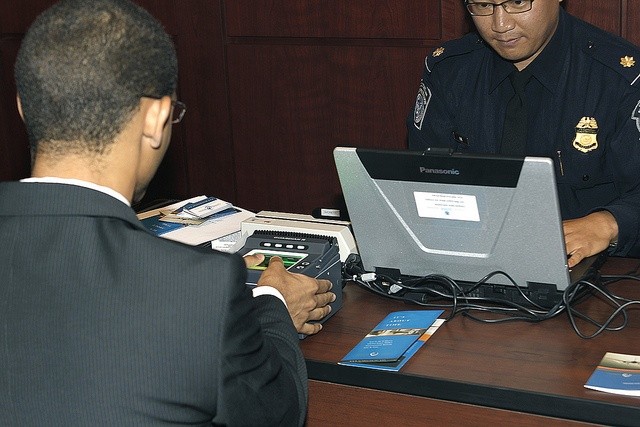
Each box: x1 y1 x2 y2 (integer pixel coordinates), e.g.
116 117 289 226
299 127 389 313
333 147 611 307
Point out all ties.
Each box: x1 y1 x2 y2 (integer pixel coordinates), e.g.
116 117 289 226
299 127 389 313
500 72 531 157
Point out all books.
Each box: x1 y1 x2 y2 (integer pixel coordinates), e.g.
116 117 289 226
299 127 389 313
337 309 446 372
135 193 257 245
584 351 640 395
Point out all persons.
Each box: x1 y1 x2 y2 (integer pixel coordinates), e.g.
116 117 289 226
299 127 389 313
0 2 336 425
406 0 639 268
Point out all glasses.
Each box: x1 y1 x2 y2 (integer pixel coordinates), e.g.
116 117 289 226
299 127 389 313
464 0 536 17
135 94 187 124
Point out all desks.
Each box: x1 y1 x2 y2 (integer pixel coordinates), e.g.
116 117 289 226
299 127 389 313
298 253 639 425
